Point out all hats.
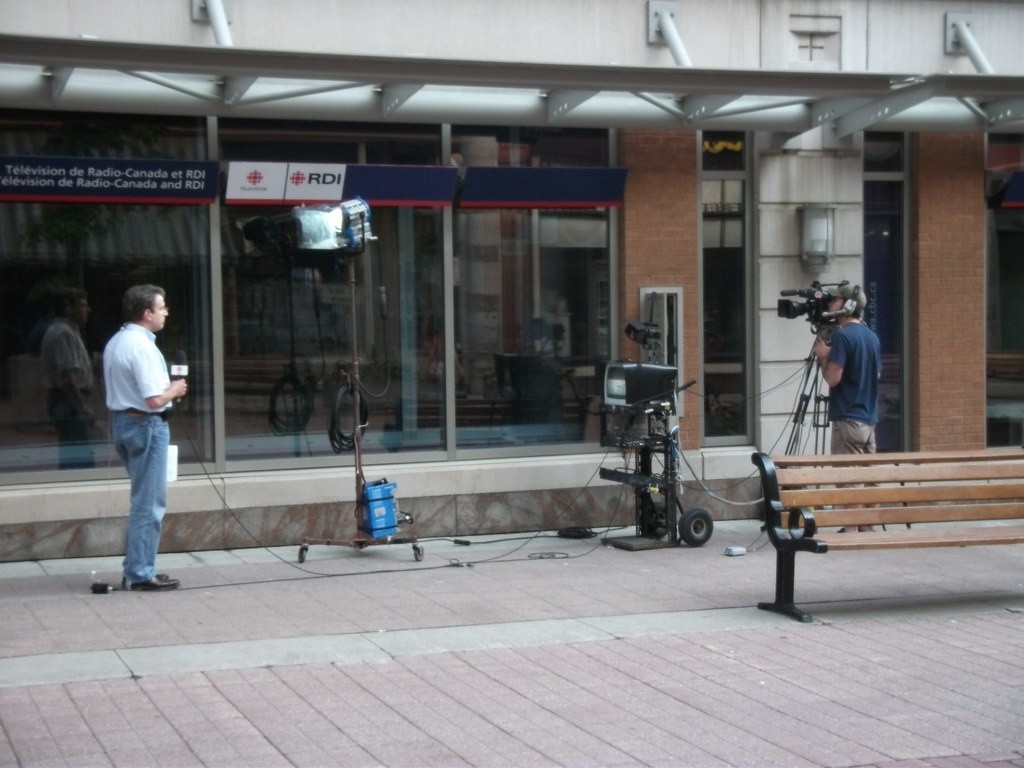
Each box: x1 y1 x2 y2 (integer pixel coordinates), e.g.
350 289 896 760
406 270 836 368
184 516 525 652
827 284 867 310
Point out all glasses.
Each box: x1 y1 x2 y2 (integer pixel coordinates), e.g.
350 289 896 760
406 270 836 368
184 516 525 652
150 306 169 312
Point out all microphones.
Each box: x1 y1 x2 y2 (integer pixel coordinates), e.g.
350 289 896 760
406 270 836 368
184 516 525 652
170 350 188 403
781 289 802 296
822 310 846 317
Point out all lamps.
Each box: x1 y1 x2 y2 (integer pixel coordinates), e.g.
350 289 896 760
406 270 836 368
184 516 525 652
796 202 835 265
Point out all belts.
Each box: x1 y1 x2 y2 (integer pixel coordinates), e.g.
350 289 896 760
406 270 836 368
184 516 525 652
127 408 164 417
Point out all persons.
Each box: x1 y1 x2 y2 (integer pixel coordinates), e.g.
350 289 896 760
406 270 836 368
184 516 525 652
33 293 95 469
103 284 188 591
812 284 882 533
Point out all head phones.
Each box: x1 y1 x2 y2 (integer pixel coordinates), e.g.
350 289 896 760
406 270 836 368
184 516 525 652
843 285 860 316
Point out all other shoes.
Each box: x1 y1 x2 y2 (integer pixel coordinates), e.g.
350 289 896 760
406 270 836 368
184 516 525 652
837 526 876 534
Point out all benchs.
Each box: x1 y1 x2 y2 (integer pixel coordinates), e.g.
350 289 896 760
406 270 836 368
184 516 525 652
752 446 1024 622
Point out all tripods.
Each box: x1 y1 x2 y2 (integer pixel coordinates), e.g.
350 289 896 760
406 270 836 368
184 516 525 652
784 325 842 456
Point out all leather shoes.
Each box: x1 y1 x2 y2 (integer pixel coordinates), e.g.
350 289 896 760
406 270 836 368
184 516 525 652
132 577 180 590
121 574 169 589
